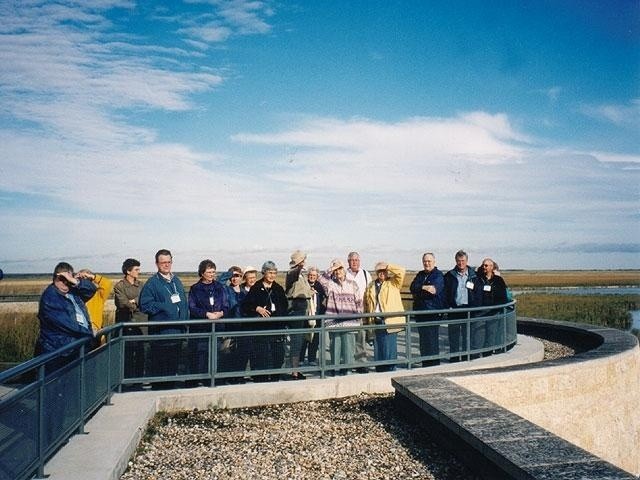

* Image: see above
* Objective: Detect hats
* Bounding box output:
[243,267,257,279]
[288,250,305,268]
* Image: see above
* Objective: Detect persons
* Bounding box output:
[189,259,230,386]
[410,253,445,366]
[316,258,363,377]
[138,249,188,389]
[73,269,113,348]
[299,267,326,366]
[346,252,377,373]
[445,249,513,363]
[114,259,145,387]
[242,261,288,381]
[285,249,316,380]
[365,262,407,371]
[217,266,249,384]
[33,262,97,374]
[240,270,258,295]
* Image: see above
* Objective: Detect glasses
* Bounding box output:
[232,274,242,278]
[481,264,491,268]
